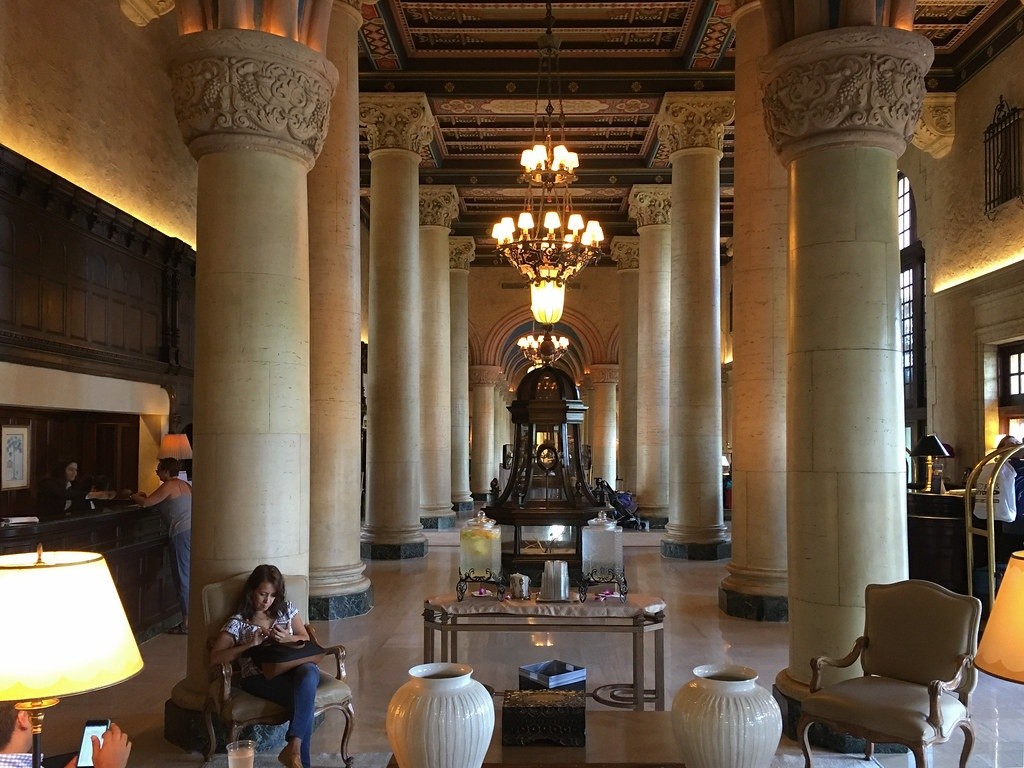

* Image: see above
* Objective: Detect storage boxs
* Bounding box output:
[500,689,587,748]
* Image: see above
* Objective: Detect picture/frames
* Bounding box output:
[2,424,32,490]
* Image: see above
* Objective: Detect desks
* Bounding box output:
[422,593,666,712]
[907,488,981,594]
[386,707,686,768]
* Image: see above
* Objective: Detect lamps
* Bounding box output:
[0,542,144,768]
[972,550,1023,685]
[157,434,193,461]
[490,2,604,365]
[910,434,950,493]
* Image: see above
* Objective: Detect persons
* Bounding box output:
[38,457,87,519]
[0,701,132,768]
[209,565,321,768]
[129,457,192,635]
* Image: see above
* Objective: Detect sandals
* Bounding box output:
[167,622,187,634]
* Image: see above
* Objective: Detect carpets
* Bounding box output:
[200,750,883,768]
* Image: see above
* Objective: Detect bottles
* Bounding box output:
[670,663,783,768]
[385,662,495,768]
[580,510,624,582]
[458,510,504,583]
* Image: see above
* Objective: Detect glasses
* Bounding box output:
[155,469,163,472]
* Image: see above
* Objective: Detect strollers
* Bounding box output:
[594,478,647,532]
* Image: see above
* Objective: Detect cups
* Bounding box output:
[226,740,256,768]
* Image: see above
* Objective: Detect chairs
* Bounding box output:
[199,571,354,768]
[796,578,983,768]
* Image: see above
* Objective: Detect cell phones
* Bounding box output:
[276,621,288,630]
[75,718,111,768]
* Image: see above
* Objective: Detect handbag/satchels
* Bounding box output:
[973,461,1016,522]
[85,475,117,508]
[242,628,327,681]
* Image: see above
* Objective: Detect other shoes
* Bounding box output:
[278,747,302,768]
